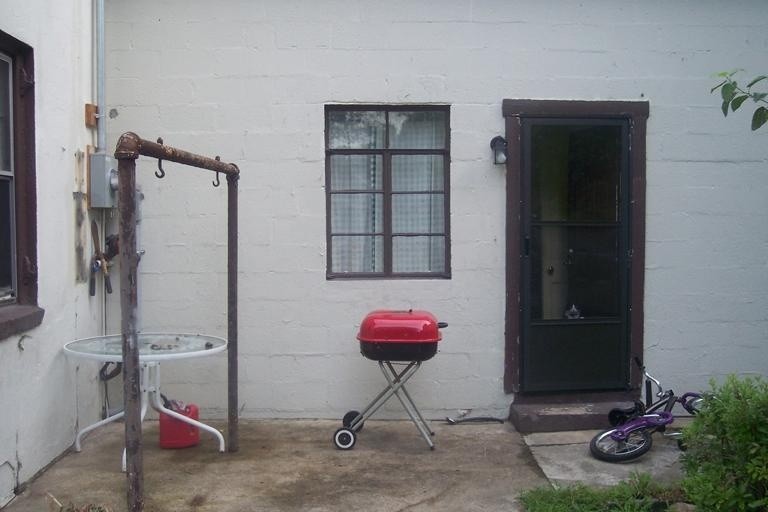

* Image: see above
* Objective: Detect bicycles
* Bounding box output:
[589,356,721,465]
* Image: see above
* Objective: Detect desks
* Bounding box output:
[64,330,228,472]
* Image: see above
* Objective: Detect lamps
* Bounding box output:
[491,135,511,165]
